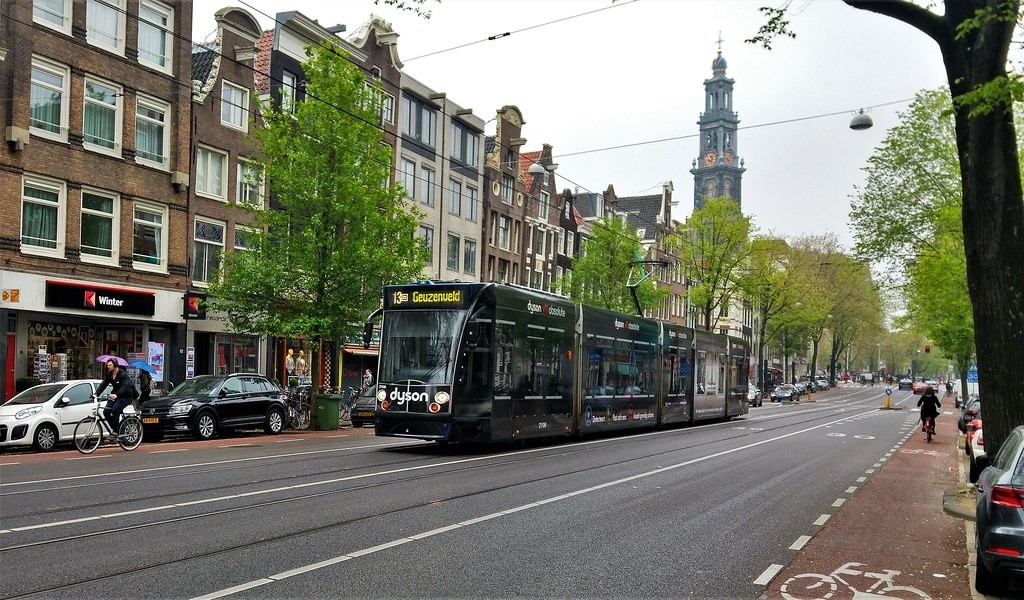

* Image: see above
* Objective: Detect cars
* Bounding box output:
[976,424,1023,596]
[350,384,376,428]
[770,375,831,403]
[957,394,987,484]
[0,379,137,454]
[747,385,763,407]
[898,377,938,394]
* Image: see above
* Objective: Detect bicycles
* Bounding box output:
[919,405,938,443]
[73,395,144,455]
[323,383,363,421]
[285,386,313,430]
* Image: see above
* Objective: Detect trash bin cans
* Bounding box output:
[314,393,343,429]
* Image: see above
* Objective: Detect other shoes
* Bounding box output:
[105,434,118,440]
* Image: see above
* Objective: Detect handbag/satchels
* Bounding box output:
[129,380,139,399]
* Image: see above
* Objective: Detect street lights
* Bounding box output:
[876,343,880,383]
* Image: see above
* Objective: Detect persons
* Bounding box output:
[946,381,952,397]
[773,375,781,387]
[138,368,152,404]
[286,349,295,377]
[921,376,925,382]
[697,382,704,394]
[363,369,374,391]
[917,386,940,435]
[90,358,135,439]
[295,350,309,377]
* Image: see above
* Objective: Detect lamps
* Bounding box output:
[528,163,544,175]
[547,163,559,173]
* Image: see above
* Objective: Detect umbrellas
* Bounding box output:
[96,355,130,366]
[128,360,157,374]
[763,367,783,382]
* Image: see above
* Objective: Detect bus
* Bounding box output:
[363,256,751,451]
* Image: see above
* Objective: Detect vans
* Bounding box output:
[950,380,979,409]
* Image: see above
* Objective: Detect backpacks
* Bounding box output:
[148,376,155,391]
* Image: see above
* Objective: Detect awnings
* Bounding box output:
[341,342,379,356]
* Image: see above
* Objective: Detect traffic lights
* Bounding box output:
[924,345,930,353]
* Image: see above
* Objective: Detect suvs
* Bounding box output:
[135,372,290,442]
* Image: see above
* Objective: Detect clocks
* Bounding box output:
[704,151,717,167]
[724,153,733,164]
